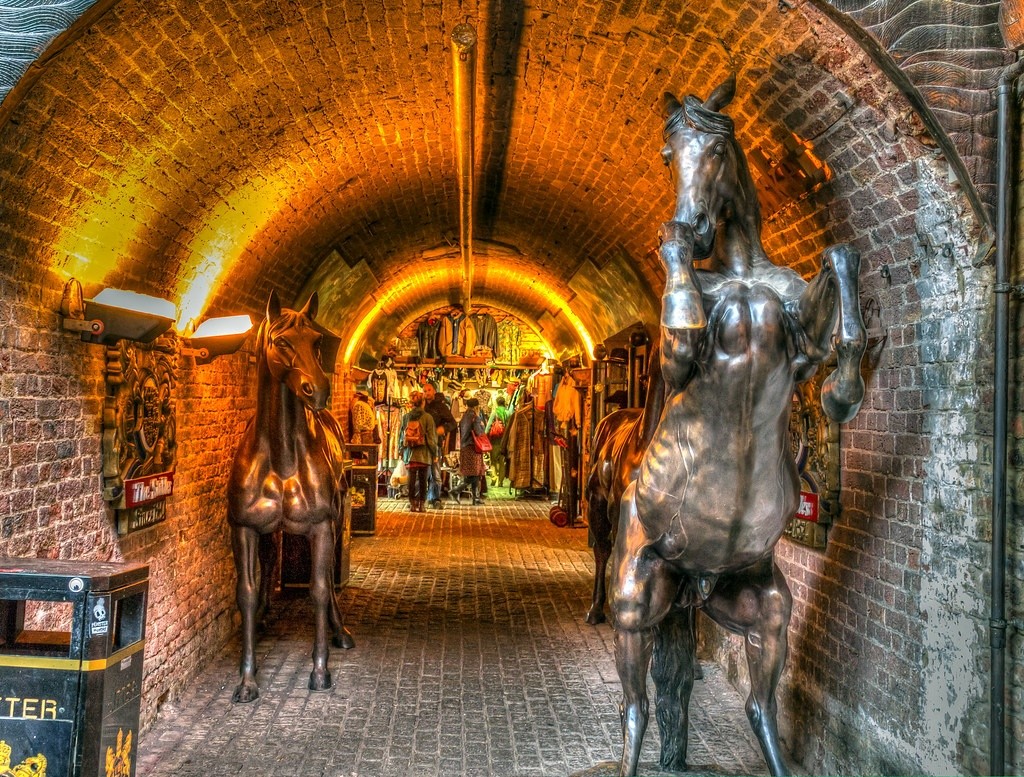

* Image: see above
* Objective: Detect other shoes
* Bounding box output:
[491,478,496,486]
[409,500,416,512]
[419,500,425,512]
[432,501,441,510]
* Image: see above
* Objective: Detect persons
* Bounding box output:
[423,383,456,509]
[475,388,491,414]
[399,391,439,512]
[349,391,382,445]
[448,398,486,505]
[486,396,509,487]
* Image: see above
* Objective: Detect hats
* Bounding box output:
[467,398,479,407]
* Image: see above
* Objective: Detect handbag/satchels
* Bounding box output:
[489,411,506,438]
[470,430,493,454]
[391,459,409,487]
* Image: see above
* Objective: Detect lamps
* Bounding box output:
[179,314,255,366]
[65,285,177,349]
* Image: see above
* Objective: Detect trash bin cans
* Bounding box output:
[281,460,352,589]
[345,443,379,536]
[0,555,150,777]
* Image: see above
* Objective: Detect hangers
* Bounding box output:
[429,313,438,320]
[452,309,461,314]
[564,367,569,377]
[478,307,485,314]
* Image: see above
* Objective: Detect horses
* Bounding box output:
[225,287,358,702]
[586,68,867,777]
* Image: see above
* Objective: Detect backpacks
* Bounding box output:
[403,414,426,448]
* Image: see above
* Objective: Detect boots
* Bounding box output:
[473,486,485,505]
[448,481,468,504]
